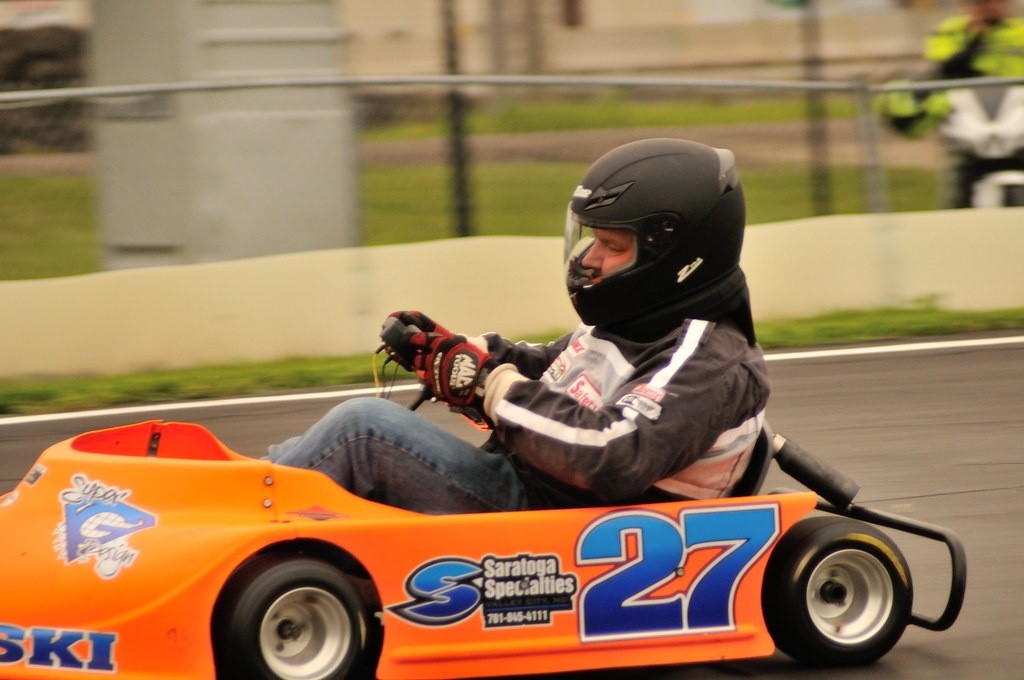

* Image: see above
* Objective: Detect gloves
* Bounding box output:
[379,310,488,393]
[408,331,502,431]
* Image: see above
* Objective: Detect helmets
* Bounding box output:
[563,137,746,326]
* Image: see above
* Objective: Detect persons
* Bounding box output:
[255,137,773,518]
[877,0,1024,211]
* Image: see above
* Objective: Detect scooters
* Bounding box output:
[905,80,1024,211]
